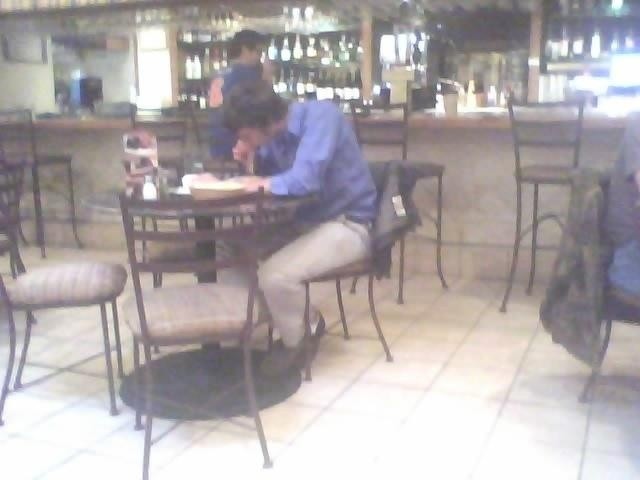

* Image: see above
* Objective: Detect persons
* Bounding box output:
[223,78,380,373]
[599,110,640,294]
[207,28,282,169]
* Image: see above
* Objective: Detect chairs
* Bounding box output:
[260,159,394,382]
[119,186,273,479]
[0,160,126,416]
[577,168,638,402]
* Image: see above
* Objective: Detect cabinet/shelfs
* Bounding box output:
[175,2,360,108]
[434,16,640,119]
[168,0,640,107]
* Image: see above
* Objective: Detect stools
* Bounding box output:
[499,102,585,313]
[129,106,259,263]
[3,109,83,258]
[350,105,449,305]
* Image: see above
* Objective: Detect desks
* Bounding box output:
[82,178,322,421]
